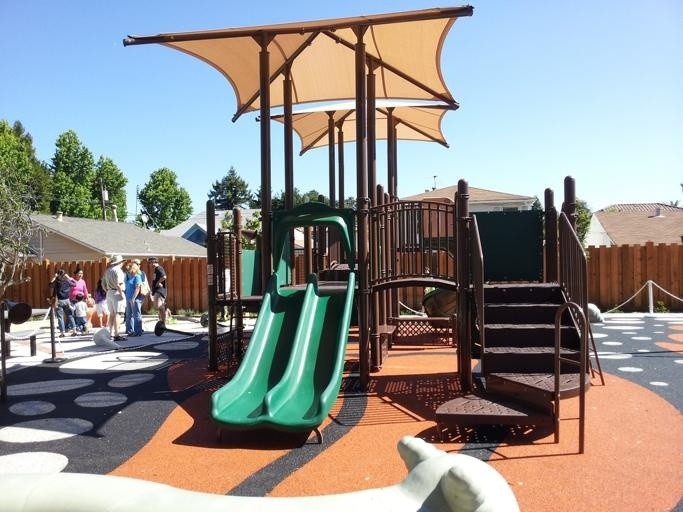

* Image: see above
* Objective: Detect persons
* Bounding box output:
[48,269,77,338]
[64,269,88,332]
[66,292,88,335]
[148,257,166,325]
[92,254,151,342]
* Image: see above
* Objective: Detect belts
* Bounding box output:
[107,288,121,291]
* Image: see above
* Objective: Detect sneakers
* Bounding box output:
[58,327,90,339]
[109,329,142,342]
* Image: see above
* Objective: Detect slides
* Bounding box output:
[210,271,356,429]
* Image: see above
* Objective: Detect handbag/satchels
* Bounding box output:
[51,295,61,309]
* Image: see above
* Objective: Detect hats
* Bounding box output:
[147,257,160,263]
[109,252,125,267]
